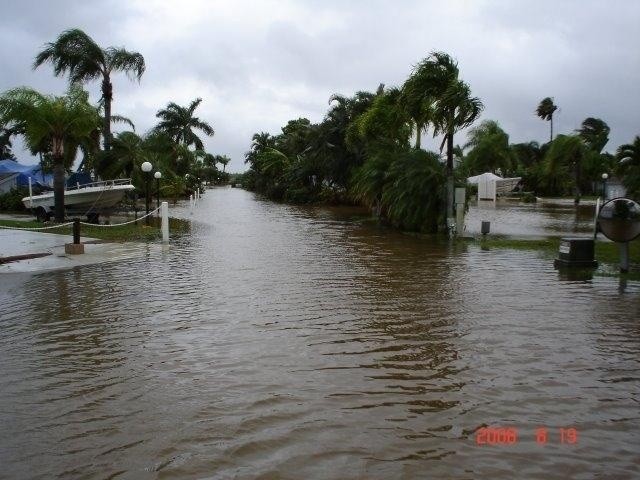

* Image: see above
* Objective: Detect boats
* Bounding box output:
[21,177,135,224]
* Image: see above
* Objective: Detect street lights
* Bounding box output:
[154,172,162,220]
[601,173,609,204]
[185,174,190,199]
[140,162,154,226]
